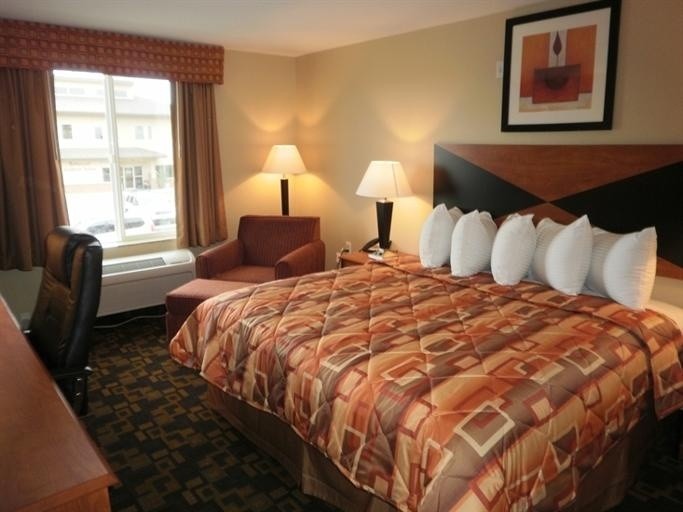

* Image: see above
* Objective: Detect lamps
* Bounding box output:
[262,145,308,217]
[355,160,413,253]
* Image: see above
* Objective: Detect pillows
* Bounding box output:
[419,203,659,314]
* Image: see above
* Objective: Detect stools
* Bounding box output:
[166,278,244,348]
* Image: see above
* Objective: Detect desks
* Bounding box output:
[1,293,121,510]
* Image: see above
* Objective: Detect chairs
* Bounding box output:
[195,215,325,283]
[23,225,103,416]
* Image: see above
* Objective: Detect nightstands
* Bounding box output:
[340,250,418,268]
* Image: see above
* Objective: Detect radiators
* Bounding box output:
[95,248,196,318]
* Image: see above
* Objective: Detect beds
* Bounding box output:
[208,143,682,512]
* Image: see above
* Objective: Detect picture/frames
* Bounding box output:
[500,1,622,133]
